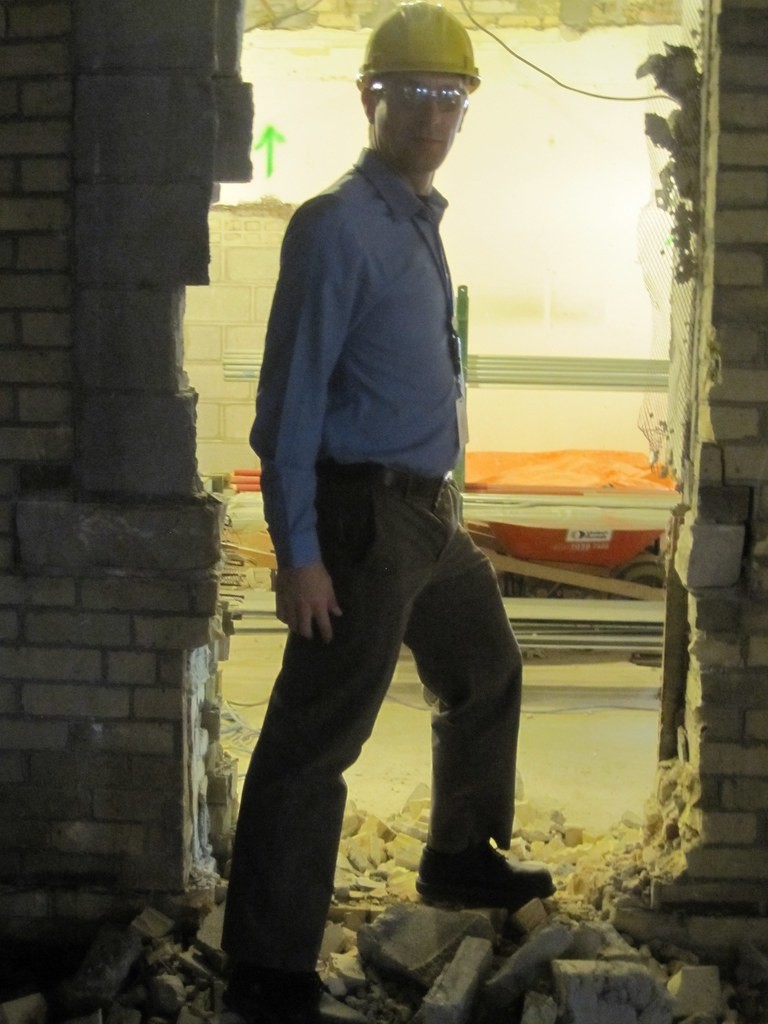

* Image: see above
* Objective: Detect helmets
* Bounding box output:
[363,4,480,93]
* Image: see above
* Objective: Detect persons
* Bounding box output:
[222,2,556,1024]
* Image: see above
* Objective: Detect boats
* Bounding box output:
[227,471,681,580]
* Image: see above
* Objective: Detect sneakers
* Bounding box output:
[415,841,556,905]
[221,963,322,1024]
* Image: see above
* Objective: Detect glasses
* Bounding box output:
[369,81,470,112]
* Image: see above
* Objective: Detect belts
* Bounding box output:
[317,460,442,498]
